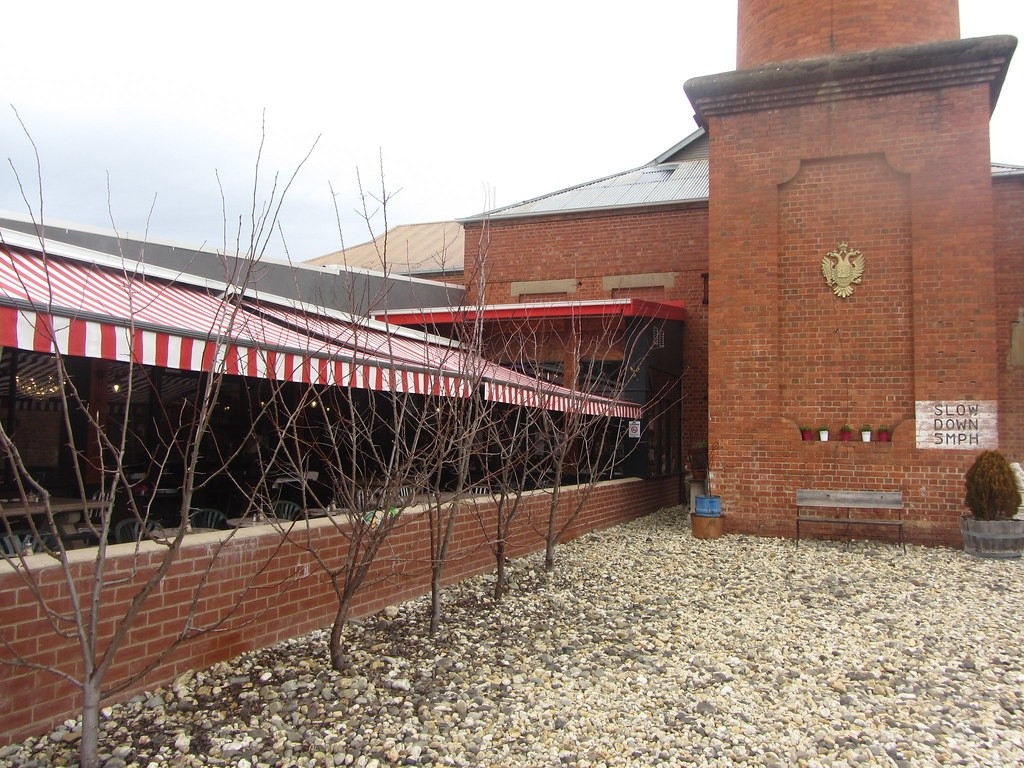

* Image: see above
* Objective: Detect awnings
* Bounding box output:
[0,243,471,397]
[233,299,643,418]
[368,297,684,326]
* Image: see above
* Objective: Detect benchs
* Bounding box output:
[795,490,907,555]
[54,531,112,545]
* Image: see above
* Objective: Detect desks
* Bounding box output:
[390,492,479,506]
[0,498,115,552]
[226,517,292,528]
[306,508,351,516]
[149,527,220,541]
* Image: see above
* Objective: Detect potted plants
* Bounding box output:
[689,439,708,479]
[801,426,813,441]
[840,425,854,441]
[876,424,891,442]
[815,424,832,441]
[695,442,721,517]
[860,425,874,442]
[958,449,1024,558]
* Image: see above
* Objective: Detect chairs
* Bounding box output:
[190,509,233,530]
[333,485,412,508]
[472,485,491,494]
[270,500,306,521]
[113,518,163,545]
